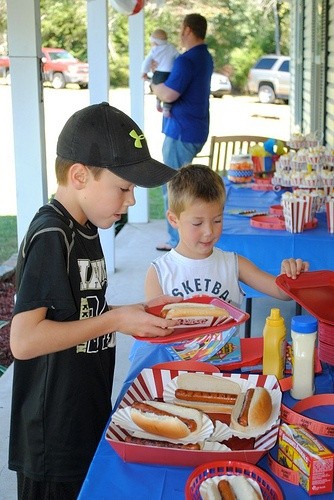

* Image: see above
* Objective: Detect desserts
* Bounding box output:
[271,132,334,187]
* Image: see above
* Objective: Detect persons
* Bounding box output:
[145,164,309,338]
[7,102,184,500]
[150,14,214,251]
[140,30,182,117]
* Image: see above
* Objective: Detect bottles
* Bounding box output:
[227,155,254,183]
[290,316,317,400]
[263,307,287,380]
[250,139,285,173]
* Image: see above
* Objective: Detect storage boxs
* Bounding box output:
[104,368,282,467]
[275,424,334,495]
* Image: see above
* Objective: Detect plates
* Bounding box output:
[129,296,250,344]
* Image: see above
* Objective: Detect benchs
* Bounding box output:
[207,135,289,177]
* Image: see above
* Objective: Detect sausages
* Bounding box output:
[124,435,200,451]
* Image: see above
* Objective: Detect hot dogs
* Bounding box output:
[231,387,272,431]
[174,372,242,414]
[205,474,261,500]
[160,303,229,319]
[131,401,203,437]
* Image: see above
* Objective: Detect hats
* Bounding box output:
[56,102,179,189]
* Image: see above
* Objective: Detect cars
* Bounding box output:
[210,71,232,99]
[247,53,292,104]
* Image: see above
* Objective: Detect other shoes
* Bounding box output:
[155,244,171,251]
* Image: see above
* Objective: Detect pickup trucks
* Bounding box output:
[0,47,88,90]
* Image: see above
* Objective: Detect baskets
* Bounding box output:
[184,460,284,500]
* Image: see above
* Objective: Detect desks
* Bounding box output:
[75,336,334,500]
[218,175,334,339]
